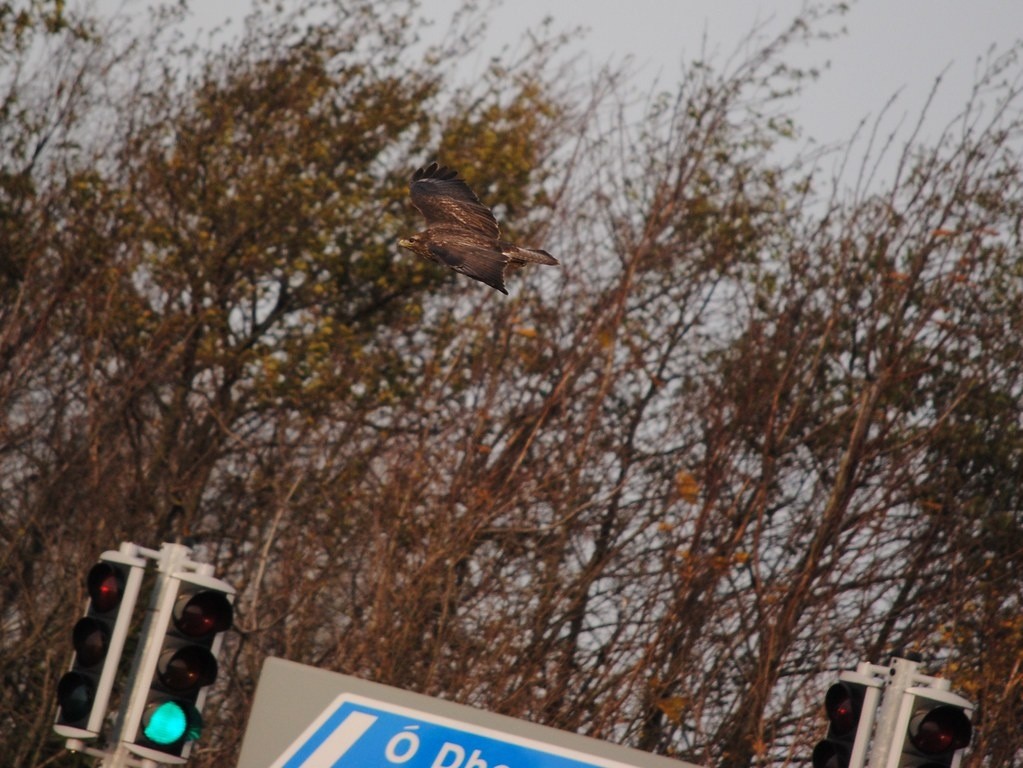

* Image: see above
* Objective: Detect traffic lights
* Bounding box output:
[122,570,239,764]
[51,549,148,739]
[809,671,884,768]
[884,688,977,768]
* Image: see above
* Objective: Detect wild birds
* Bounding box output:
[397,162,561,298]
